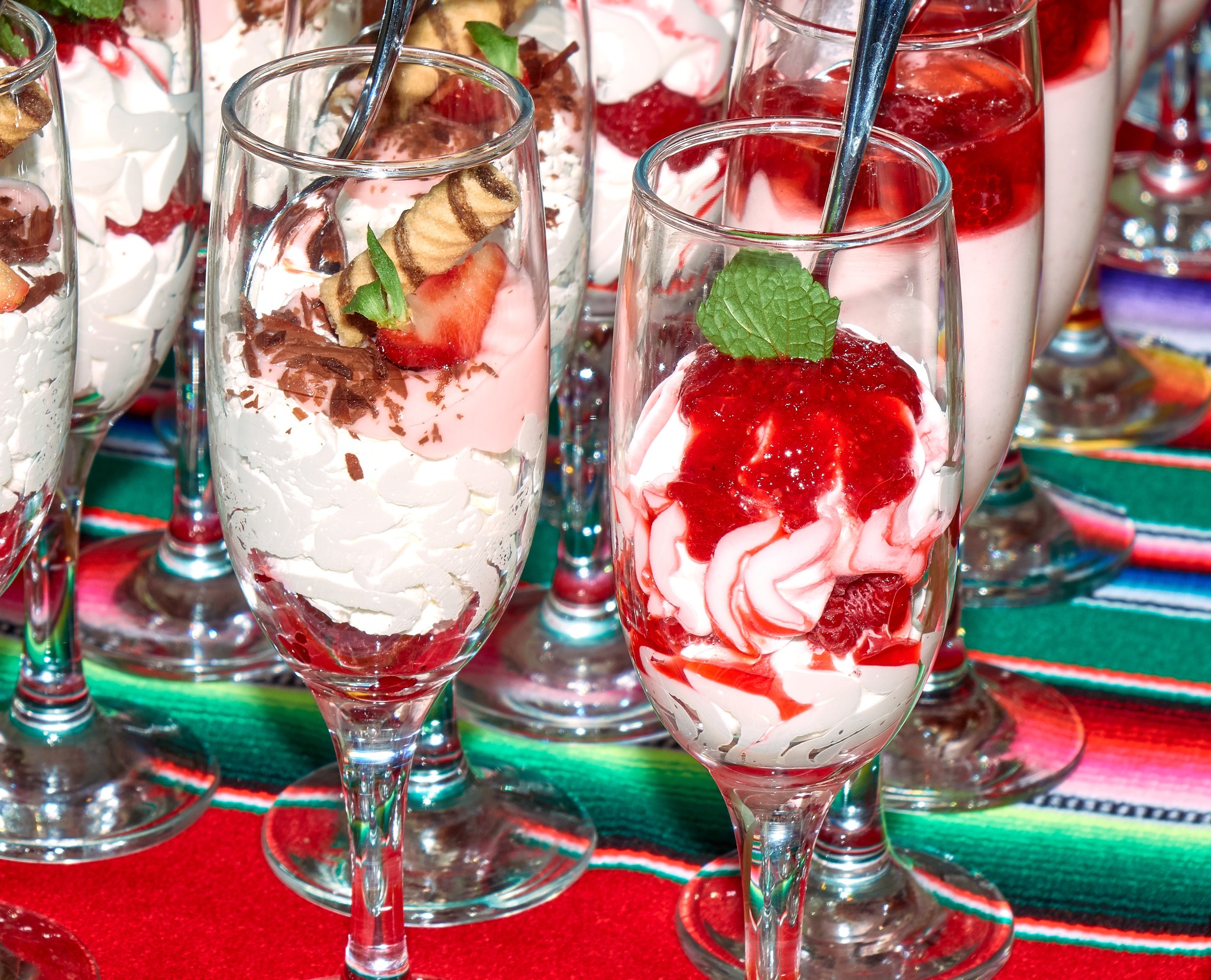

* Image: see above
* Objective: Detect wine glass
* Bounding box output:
[0,0,1211,980]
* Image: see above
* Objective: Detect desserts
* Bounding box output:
[0,0,1191,788]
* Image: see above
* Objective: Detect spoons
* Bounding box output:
[243,0,414,329]
